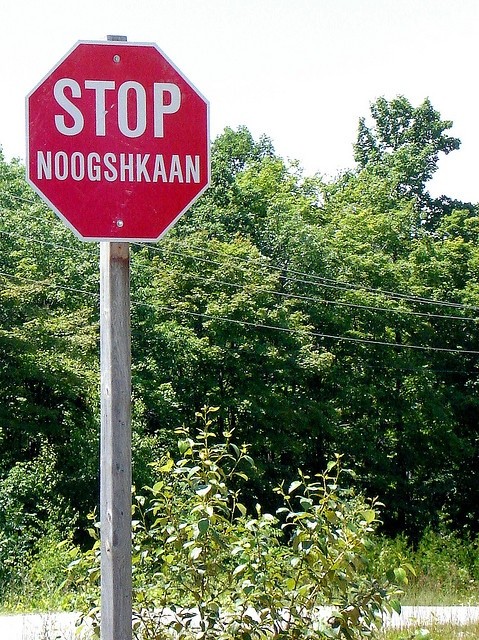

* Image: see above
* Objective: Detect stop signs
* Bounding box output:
[25,39,211,244]
